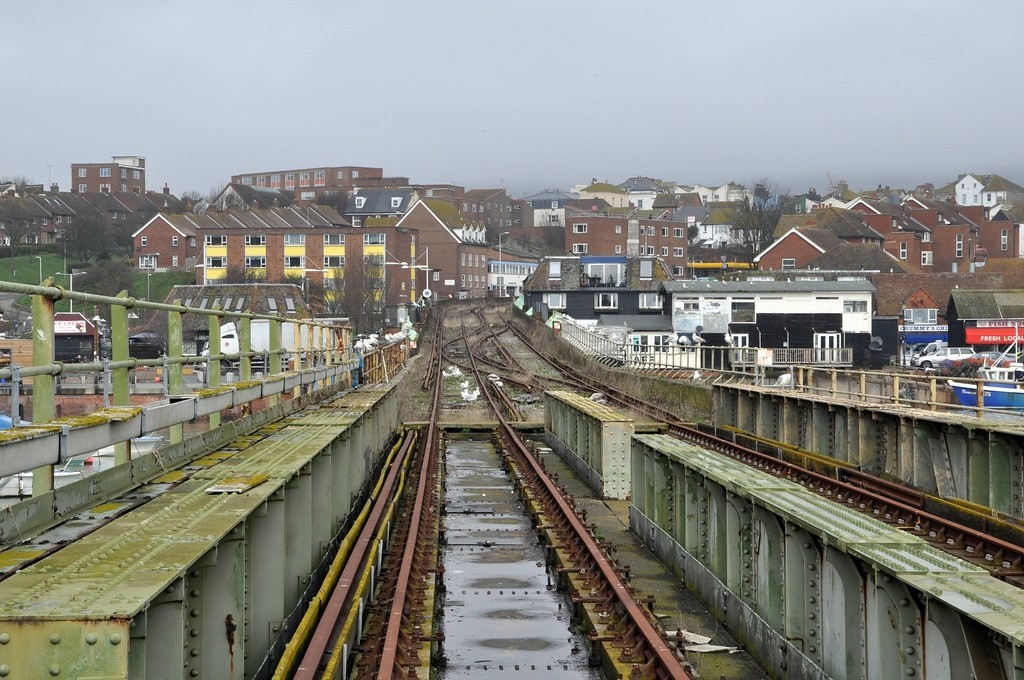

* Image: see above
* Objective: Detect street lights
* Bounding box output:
[380,262,408,266]
[148,253,160,301]
[56,272,86,312]
[401,247,428,289]
[899,301,907,367]
[36,256,42,283]
[302,252,329,291]
[499,232,509,297]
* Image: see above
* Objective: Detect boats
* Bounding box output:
[947,321,1024,409]
[92,436,163,457]
[0,472,81,496]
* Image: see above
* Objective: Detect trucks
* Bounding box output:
[910,343,940,366]
[202,318,350,374]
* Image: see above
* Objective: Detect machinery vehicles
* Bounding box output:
[379,302,420,338]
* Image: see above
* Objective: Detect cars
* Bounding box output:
[914,348,977,368]
[968,351,1014,368]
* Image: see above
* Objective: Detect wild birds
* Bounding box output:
[680,263,820,289]
[561,312,621,344]
[353,315,413,354]
[589,391,607,405]
[725,332,737,347]
[693,370,702,382]
[774,373,791,388]
[663,330,706,347]
[442,367,480,403]
